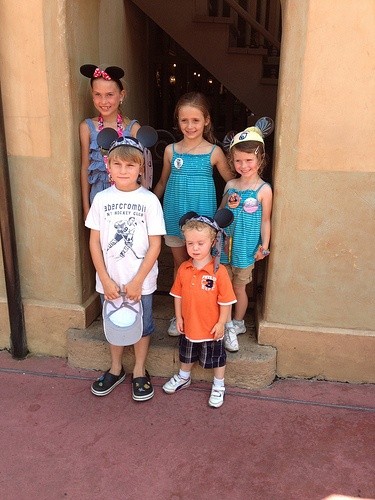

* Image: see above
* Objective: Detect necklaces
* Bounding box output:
[174,139,204,169]
[98,110,123,187]
[227,177,258,208]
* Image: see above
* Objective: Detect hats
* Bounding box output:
[222,116,274,150]
[97,124,158,154]
[101,285,144,346]
[177,208,233,238]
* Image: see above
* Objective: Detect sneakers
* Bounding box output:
[162,373,192,395]
[208,382,226,407]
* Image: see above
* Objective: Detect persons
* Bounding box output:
[162,207,237,408]
[216,117,274,335]
[85,126,167,401]
[79,64,141,221]
[154,91,239,352]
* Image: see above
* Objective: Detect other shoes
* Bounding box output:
[233,320,247,336]
[167,316,182,337]
[223,326,240,353]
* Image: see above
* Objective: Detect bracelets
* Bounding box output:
[257,244,270,256]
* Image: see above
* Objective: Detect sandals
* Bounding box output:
[131,369,155,402]
[90,363,126,397]
